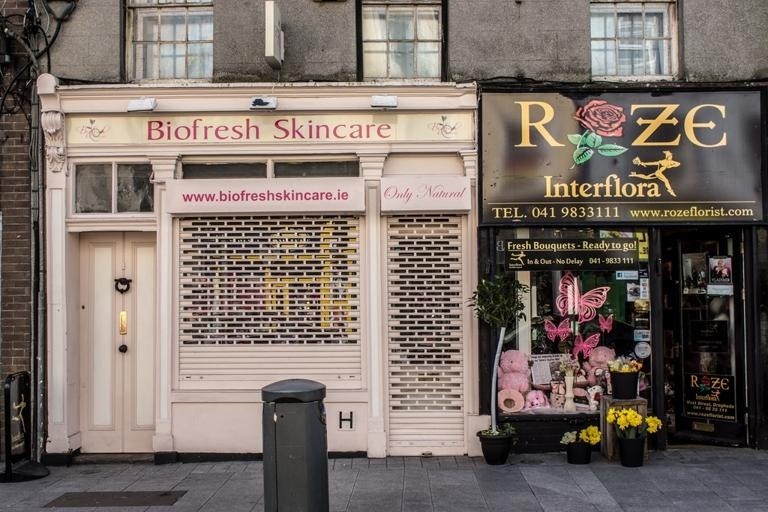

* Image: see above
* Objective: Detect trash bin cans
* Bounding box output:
[261,379,329,512]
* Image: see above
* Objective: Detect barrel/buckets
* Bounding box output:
[618,438,646,468]
[610,370,639,399]
[567,441,591,464]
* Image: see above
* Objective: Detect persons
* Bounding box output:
[712,258,731,282]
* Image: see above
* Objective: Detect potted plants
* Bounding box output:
[464,272,530,466]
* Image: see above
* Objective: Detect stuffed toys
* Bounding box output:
[498,346,615,412]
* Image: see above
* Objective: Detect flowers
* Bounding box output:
[560,425,602,445]
[606,407,663,438]
[565,100,630,169]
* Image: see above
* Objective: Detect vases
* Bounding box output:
[567,442,592,463]
[616,436,645,467]
